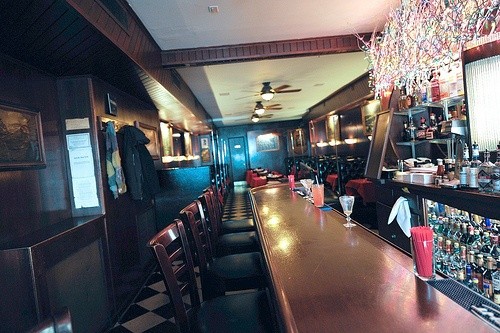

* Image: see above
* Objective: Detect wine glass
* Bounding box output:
[300,179,315,199]
[339,196,355,228]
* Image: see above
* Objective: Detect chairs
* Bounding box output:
[146,167,284,333]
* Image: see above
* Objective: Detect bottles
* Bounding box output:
[436,143,500,198]
[399,67,467,141]
[427,200,500,306]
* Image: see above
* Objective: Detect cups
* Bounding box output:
[311,184,324,207]
[289,175,295,191]
[410,237,436,282]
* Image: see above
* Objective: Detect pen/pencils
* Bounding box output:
[400,160,403,172]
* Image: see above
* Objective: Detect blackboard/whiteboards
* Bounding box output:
[364,110,393,179]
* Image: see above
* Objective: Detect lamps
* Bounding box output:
[251,114,259,123]
[260,82,276,101]
[255,101,265,114]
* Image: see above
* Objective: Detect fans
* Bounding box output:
[235,114,273,122]
[226,101,283,115]
[234,82,302,100]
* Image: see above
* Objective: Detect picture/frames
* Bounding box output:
[0,102,47,172]
[135,121,160,160]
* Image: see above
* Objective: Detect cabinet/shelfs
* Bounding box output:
[376,97,500,308]
